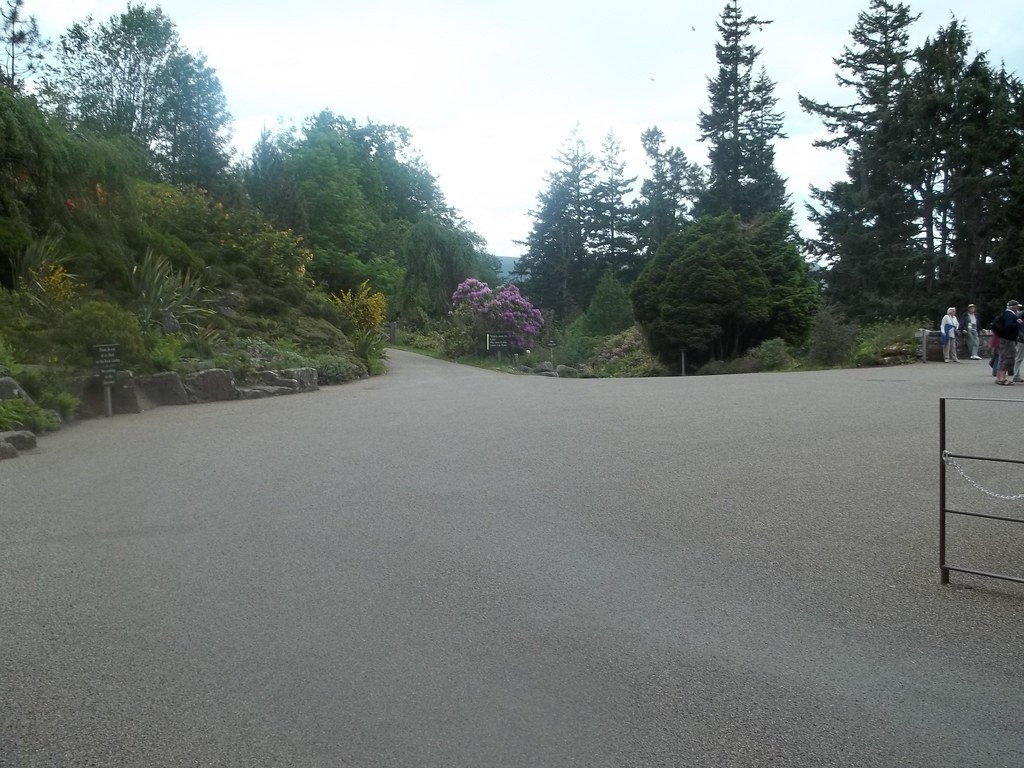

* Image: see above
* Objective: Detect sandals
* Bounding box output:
[998,379,1015,385]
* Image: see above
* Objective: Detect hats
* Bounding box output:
[1007,300,1022,307]
[968,304,976,308]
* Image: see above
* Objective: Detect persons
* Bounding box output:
[989,300,1024,385]
[960,304,985,360]
[940,307,961,363]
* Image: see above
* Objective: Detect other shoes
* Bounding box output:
[953,360,960,362]
[970,355,982,359]
[944,359,949,362]
